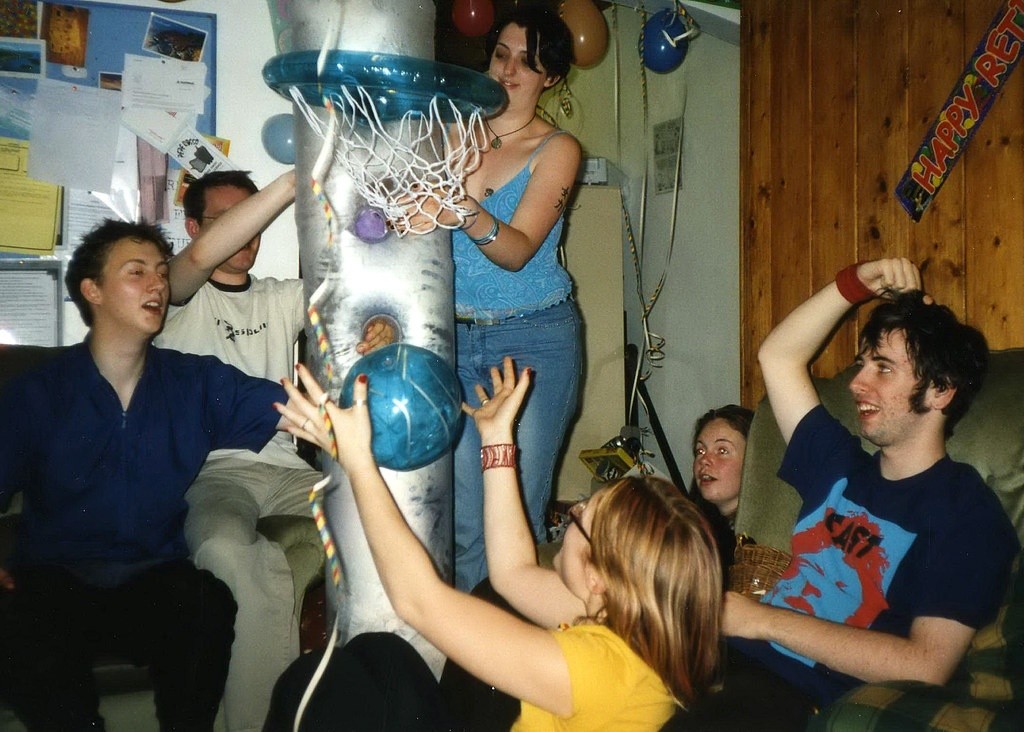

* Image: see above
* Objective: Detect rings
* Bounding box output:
[352,399,369,405]
[302,418,311,430]
[481,399,490,407]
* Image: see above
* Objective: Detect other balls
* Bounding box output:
[340,342,464,471]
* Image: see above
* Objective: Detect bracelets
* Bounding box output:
[459,201,478,230]
[470,215,499,245]
[480,444,517,473]
[835,260,876,306]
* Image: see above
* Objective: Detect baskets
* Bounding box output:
[730,544,792,603]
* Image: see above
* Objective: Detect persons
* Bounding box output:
[395,13,583,594]
[0,171,324,732]
[271,357,722,732]
[659,257,1018,732]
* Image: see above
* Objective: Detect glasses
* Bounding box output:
[568,499,591,544]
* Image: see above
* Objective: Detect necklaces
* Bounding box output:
[484,110,537,149]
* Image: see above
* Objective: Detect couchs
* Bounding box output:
[0,516,326,732]
[707,347,1024,732]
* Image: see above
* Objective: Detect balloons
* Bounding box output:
[261,113,296,164]
[452,0,494,38]
[339,343,463,474]
[558,0,608,67]
[638,10,689,75]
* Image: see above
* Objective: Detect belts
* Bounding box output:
[455,318,498,325]
